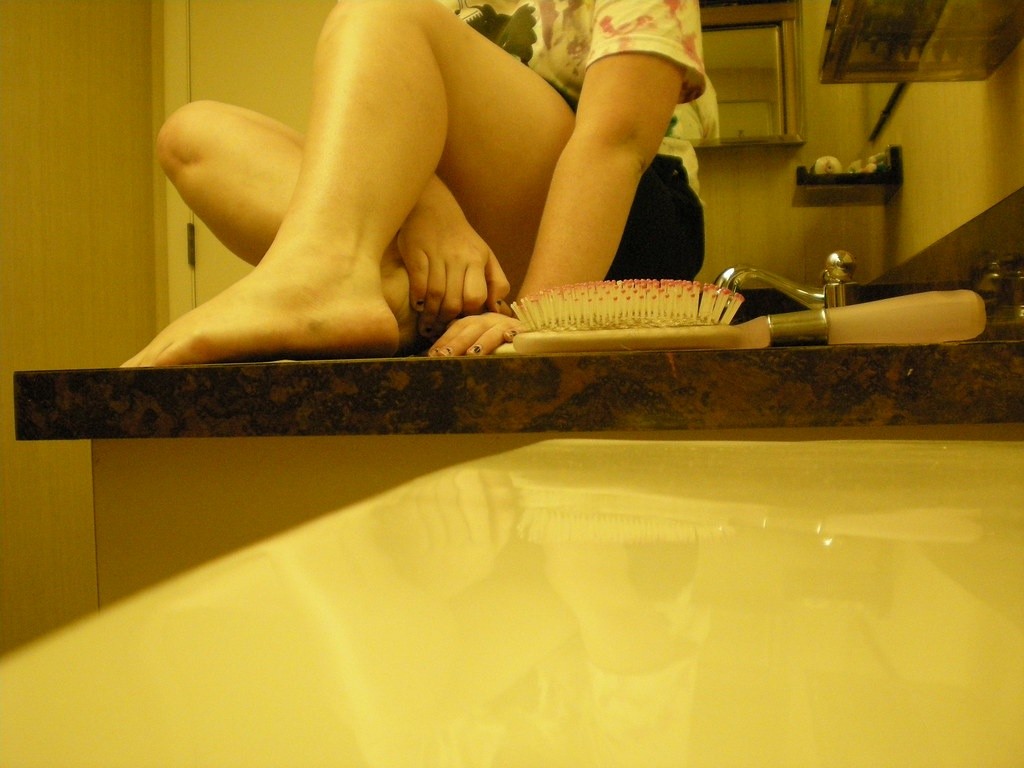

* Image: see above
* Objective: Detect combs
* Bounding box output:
[510,278,987,355]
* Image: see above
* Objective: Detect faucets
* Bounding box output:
[714,249,855,310]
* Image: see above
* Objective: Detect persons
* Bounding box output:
[119,0,705,369]
